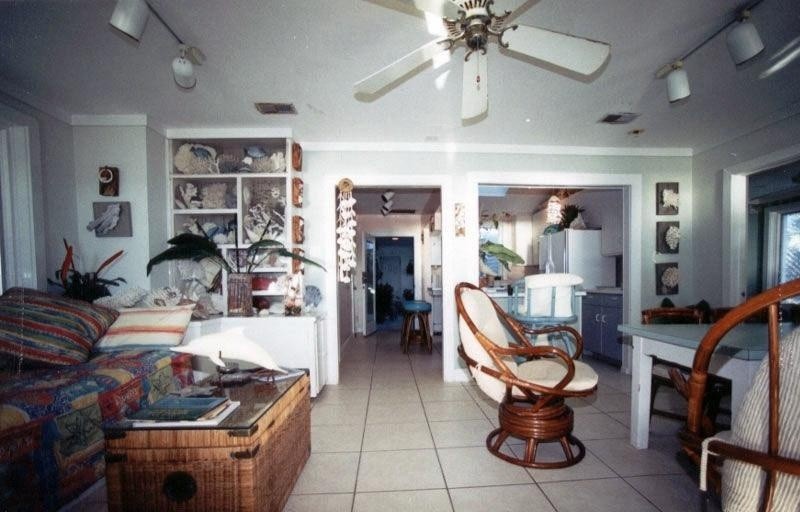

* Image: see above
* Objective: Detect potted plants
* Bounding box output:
[146,217,328,316]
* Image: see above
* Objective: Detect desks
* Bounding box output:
[106,367,313,512]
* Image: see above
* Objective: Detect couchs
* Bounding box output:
[0,346,195,511]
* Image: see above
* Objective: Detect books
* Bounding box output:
[120,392,242,427]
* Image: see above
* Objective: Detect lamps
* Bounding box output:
[547,187,563,225]
[111,1,206,89]
[381,189,395,216]
[657,0,766,103]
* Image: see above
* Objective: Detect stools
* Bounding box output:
[401,300,432,354]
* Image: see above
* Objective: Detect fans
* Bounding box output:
[352,1,612,123]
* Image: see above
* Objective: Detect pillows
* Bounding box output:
[459,288,516,402]
[0,287,120,368]
[524,274,583,316]
[93,303,196,347]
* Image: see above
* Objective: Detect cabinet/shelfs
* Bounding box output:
[166,125,293,313]
[537,228,617,291]
[581,293,622,366]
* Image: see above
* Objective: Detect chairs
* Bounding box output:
[713,304,735,434]
[451,283,600,470]
[643,307,702,427]
[507,284,583,359]
[673,277,800,511]
